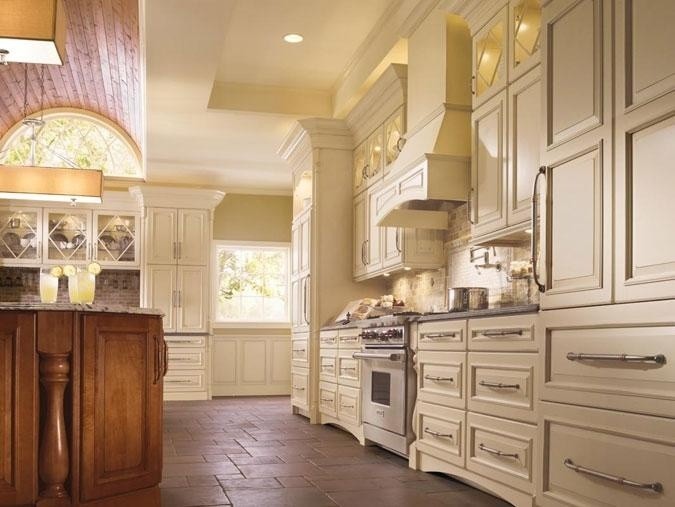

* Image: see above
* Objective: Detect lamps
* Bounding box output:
[0,0,68,66]
[0,118,104,204]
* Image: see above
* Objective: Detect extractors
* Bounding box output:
[368,110,470,230]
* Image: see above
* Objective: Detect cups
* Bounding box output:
[38,265,60,304]
[67,265,95,303]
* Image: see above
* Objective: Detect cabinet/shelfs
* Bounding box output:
[470,1,541,109]
[291,334,316,425]
[539,2,673,308]
[141,201,214,267]
[0,309,40,507]
[0,197,44,268]
[44,200,142,272]
[414,322,539,495]
[268,331,291,396]
[341,60,445,282]
[141,262,214,400]
[290,272,311,333]
[470,63,542,238]
[213,331,269,398]
[77,312,169,504]
[538,307,673,504]
[321,330,363,445]
[290,204,314,272]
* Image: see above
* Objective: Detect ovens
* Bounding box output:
[354,348,415,460]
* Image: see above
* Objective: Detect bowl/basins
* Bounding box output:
[446,287,489,311]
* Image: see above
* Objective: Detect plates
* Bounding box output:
[1,232,135,251]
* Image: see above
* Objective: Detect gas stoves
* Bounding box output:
[358,313,448,343]
